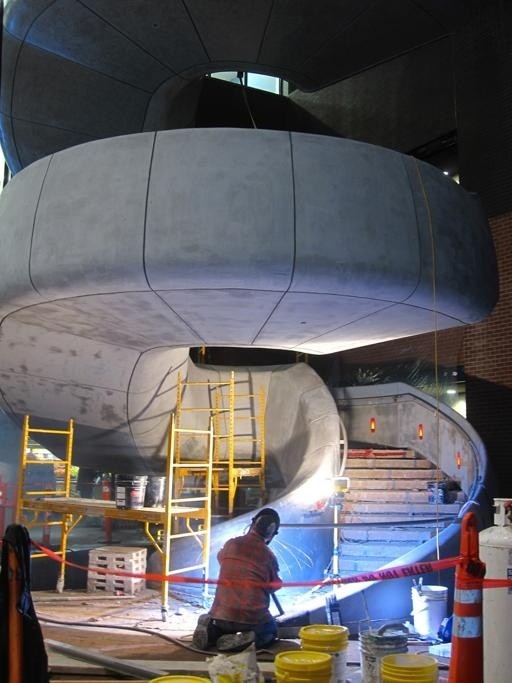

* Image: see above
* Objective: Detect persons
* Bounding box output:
[191,508,284,655]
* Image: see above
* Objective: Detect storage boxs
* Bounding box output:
[87,546,148,594]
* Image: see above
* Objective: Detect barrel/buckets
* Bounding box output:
[427,481,446,505]
[358,629,408,683]
[149,675,212,683]
[410,585,449,635]
[114,474,147,509]
[144,476,166,507]
[299,624,349,683]
[380,654,438,683]
[275,650,331,683]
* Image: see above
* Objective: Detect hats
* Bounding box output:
[252,508,280,545]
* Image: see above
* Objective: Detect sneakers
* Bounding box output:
[193,614,213,650]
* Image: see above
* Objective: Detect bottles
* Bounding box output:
[102,478,111,500]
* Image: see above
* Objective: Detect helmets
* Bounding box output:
[216,631,256,651]
